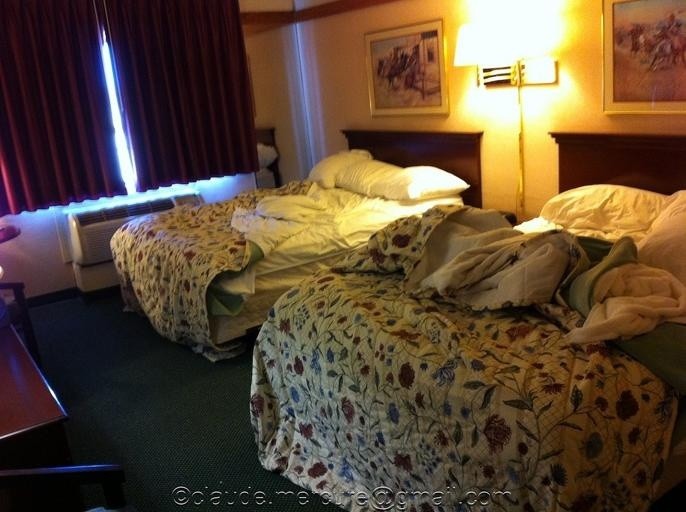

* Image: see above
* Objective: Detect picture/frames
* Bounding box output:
[597,0,686,116]
[361,16,449,119]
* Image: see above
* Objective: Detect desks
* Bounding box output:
[0,315,74,470]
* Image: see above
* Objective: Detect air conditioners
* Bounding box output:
[61,191,206,294]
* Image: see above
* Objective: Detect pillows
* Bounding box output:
[307,146,370,190]
[371,164,469,204]
[542,177,666,236]
[336,156,400,197]
[633,184,685,284]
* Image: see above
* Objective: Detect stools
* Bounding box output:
[0,280,47,367]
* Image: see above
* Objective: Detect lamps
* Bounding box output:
[452,18,536,225]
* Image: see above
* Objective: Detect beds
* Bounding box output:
[247,130,685,511]
[108,129,485,365]
